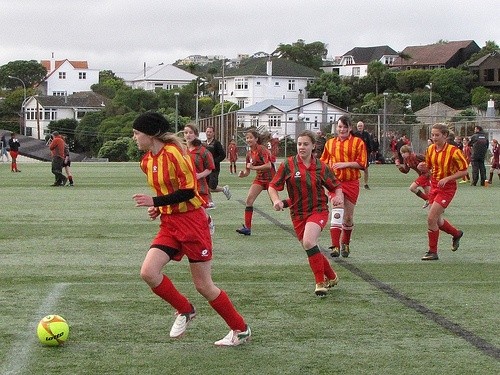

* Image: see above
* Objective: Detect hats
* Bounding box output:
[133,113,170,137]
[53,131,59,136]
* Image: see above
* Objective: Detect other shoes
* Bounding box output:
[422,200,429,208]
[364,185,370,190]
[63,178,68,186]
[459,180,466,184]
[208,216,215,235]
[11,170,14,172]
[15,170,21,172]
[485,180,492,184]
[469,183,476,186]
[51,184,59,186]
[68,182,73,186]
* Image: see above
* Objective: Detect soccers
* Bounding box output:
[36,314,69,346]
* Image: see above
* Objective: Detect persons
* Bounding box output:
[267,130,343,296]
[49,131,73,186]
[353,121,373,189]
[446,126,489,186]
[132,112,251,346]
[321,116,367,257]
[395,145,431,208]
[369,133,385,163]
[265,134,276,155]
[486,139,500,183]
[203,127,231,200]
[0,136,10,161]
[314,131,326,158]
[390,135,411,166]
[184,125,215,235]
[229,139,239,173]
[428,139,434,145]
[236,130,276,235]
[416,123,469,259]
[9,133,21,172]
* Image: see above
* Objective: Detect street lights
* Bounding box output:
[8,76,28,136]
[426,83,432,123]
[195,79,204,131]
[383,92,389,158]
[174,92,180,134]
[220,57,233,148]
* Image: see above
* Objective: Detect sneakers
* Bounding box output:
[208,202,215,209]
[224,185,231,200]
[214,326,251,346]
[328,246,340,257]
[341,243,350,258]
[315,282,328,296]
[169,304,197,338]
[324,273,339,287]
[421,251,438,260]
[236,223,251,235]
[452,230,463,251]
